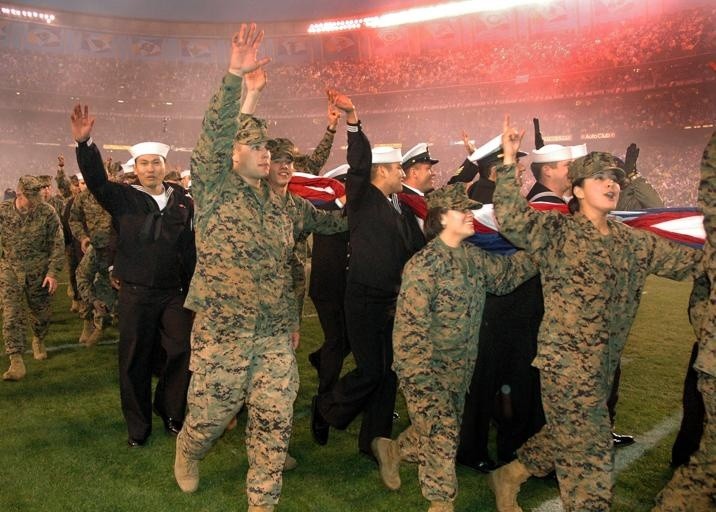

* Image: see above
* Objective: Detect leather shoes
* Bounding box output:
[393,412,400,422]
[152,402,183,437]
[310,395,330,445]
[358,434,378,464]
[309,341,325,379]
[128,433,148,447]
[544,470,559,488]
[612,433,636,448]
[671,459,687,470]
[456,456,503,474]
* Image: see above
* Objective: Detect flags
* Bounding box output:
[287,172,706,250]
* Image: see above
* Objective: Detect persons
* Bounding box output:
[0,175,66,381]
[250,48,309,156]
[308,164,351,399]
[607,143,667,447]
[239,66,349,473]
[35,150,120,347]
[311,87,413,471]
[651,133,716,512]
[392,142,439,418]
[0,50,228,191]
[291,101,343,325]
[174,23,309,512]
[310,48,457,189]
[370,182,539,512]
[71,104,196,447]
[457,8,715,207]
[496,142,587,487]
[456,134,528,472]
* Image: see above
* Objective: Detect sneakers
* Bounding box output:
[67,284,75,298]
[3,353,26,381]
[427,500,455,512]
[70,299,79,313]
[32,335,48,361]
[370,437,401,491]
[86,329,104,347]
[248,505,274,512]
[283,453,297,471]
[487,459,532,512]
[228,415,237,430]
[78,319,94,343]
[174,431,200,494]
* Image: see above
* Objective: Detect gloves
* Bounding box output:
[533,118,544,151]
[624,143,639,180]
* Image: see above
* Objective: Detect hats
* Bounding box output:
[232,113,278,150]
[469,131,529,168]
[180,170,190,178]
[265,137,294,161]
[371,147,403,164]
[109,163,120,176]
[18,175,45,206]
[425,181,482,211]
[531,144,572,164]
[74,173,84,180]
[121,158,135,173]
[323,163,350,181]
[35,176,53,187]
[563,143,589,158]
[401,142,439,170]
[127,142,170,160]
[566,152,626,186]
[164,170,181,180]
[4,188,16,200]
